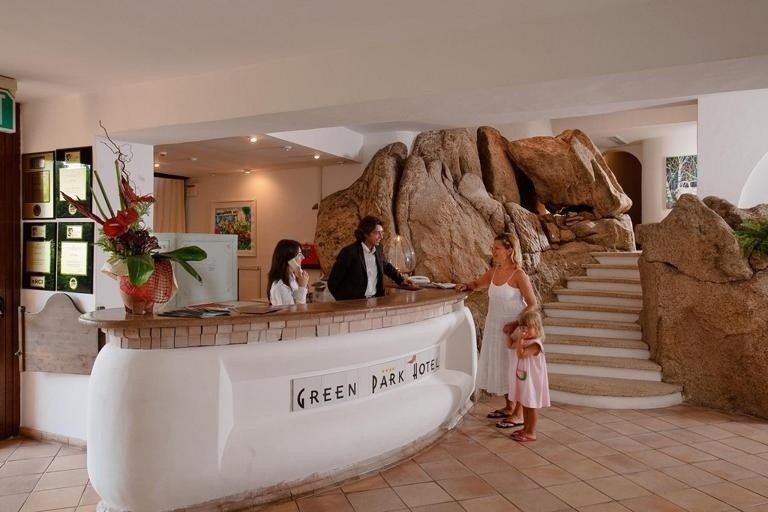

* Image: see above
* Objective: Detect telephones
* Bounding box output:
[288,258,304,278]
[408,276,430,284]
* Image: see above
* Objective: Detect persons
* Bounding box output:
[326,216,415,301]
[266,239,309,306]
[506,312,551,441]
[455,233,537,428]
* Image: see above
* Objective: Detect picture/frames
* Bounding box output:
[212,200,259,258]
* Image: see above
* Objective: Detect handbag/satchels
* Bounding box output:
[515,368,527,380]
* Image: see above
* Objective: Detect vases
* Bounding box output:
[120,275,155,314]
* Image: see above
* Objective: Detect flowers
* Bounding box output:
[58,116,210,302]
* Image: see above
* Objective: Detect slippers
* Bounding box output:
[496,418,524,428]
[487,409,512,418]
[511,429,536,441]
